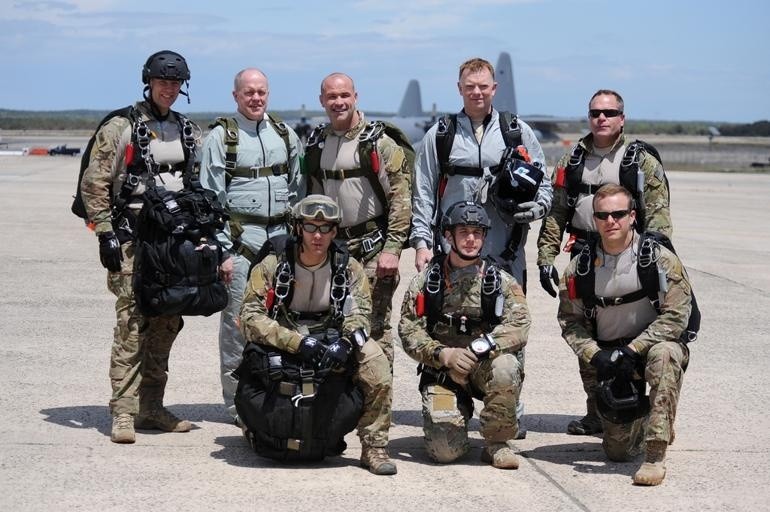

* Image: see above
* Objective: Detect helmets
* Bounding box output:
[592,371,651,424]
[441,199,493,239]
[491,159,545,217]
[141,50,191,83]
[291,194,344,237]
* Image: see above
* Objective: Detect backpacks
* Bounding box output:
[69,106,198,218]
[231,340,366,468]
[131,186,229,318]
[576,233,701,344]
[246,232,362,317]
[306,120,416,250]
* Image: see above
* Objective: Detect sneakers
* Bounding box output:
[568,413,604,436]
[111,412,137,443]
[516,419,527,439]
[480,441,520,469]
[134,404,193,432]
[633,445,668,486]
[360,444,398,475]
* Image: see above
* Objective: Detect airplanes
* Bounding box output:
[279,53,541,149]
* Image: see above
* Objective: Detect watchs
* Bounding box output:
[352,328,367,352]
[469,338,490,360]
[609,348,623,364]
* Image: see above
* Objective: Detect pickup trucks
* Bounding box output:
[48,145,81,156]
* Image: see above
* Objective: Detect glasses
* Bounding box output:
[593,209,632,221]
[588,108,624,119]
[300,219,337,234]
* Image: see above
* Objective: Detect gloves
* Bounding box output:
[318,338,354,372]
[607,345,641,380]
[446,368,470,385]
[299,335,327,365]
[98,233,125,273]
[590,350,613,381]
[539,263,560,299]
[438,347,478,375]
[512,200,547,225]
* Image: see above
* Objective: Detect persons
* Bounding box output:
[397,198,533,473]
[293,115,312,154]
[556,182,694,486]
[300,70,417,453]
[197,67,306,419]
[234,193,398,476]
[72,48,205,443]
[409,57,554,441]
[535,89,673,435]
